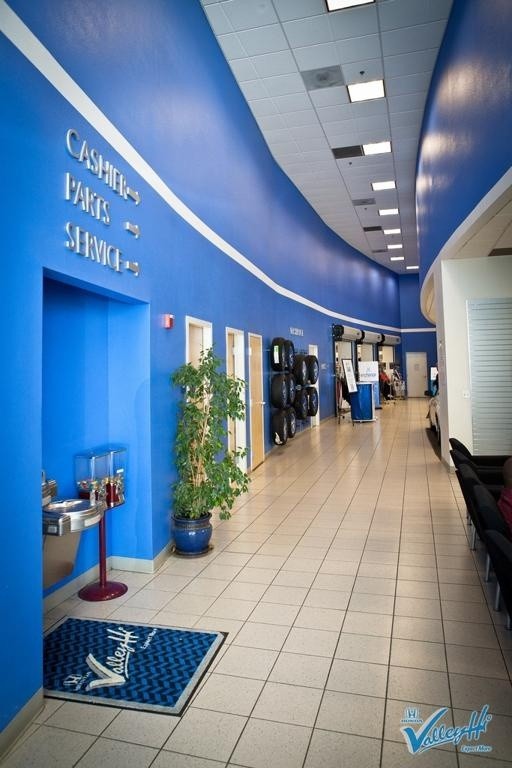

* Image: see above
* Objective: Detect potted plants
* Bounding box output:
[172,342,252,557]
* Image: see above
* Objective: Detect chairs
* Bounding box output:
[448,438,512,632]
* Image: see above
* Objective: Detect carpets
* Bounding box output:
[44,614,229,717]
